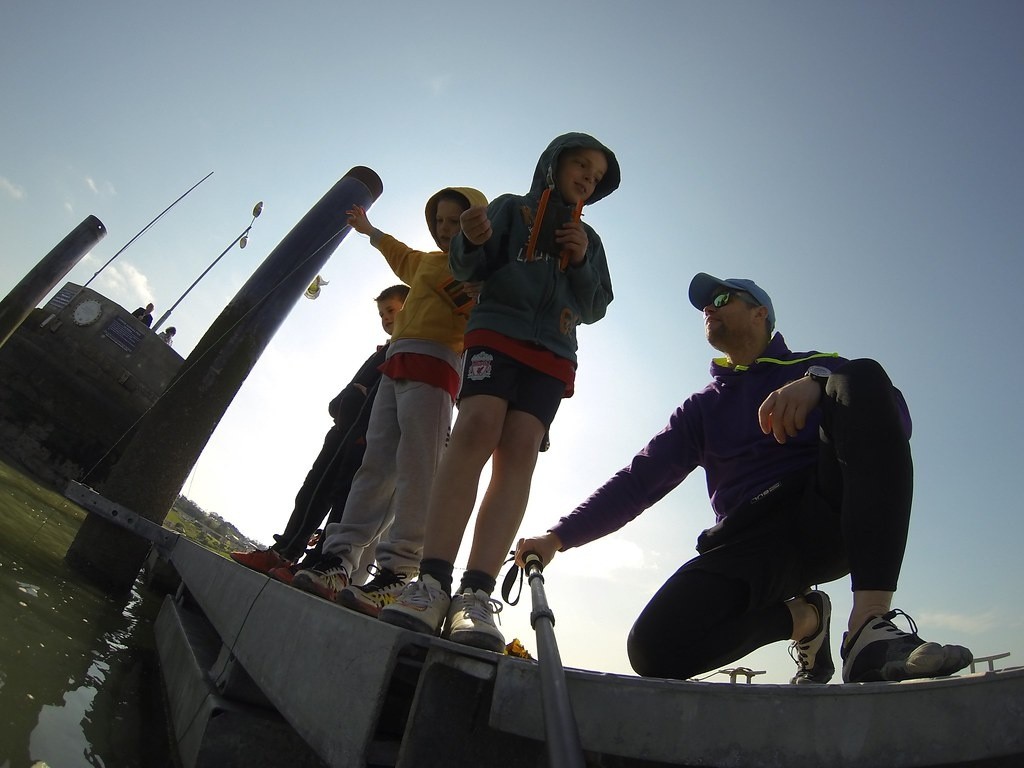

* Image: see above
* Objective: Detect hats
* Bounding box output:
[688,272,776,331]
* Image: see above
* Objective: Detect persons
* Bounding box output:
[514,273,975,684]
[293,186,489,618]
[374,132,622,651]
[229,285,410,586]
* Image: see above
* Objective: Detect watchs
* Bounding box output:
[803,364,831,383]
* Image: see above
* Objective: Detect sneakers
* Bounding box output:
[335,564,411,617]
[441,587,507,653]
[840,610,973,685]
[268,562,306,583]
[230,548,290,574]
[294,552,353,600]
[788,591,836,685]
[379,574,451,634]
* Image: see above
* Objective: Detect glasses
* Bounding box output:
[702,291,731,319]
